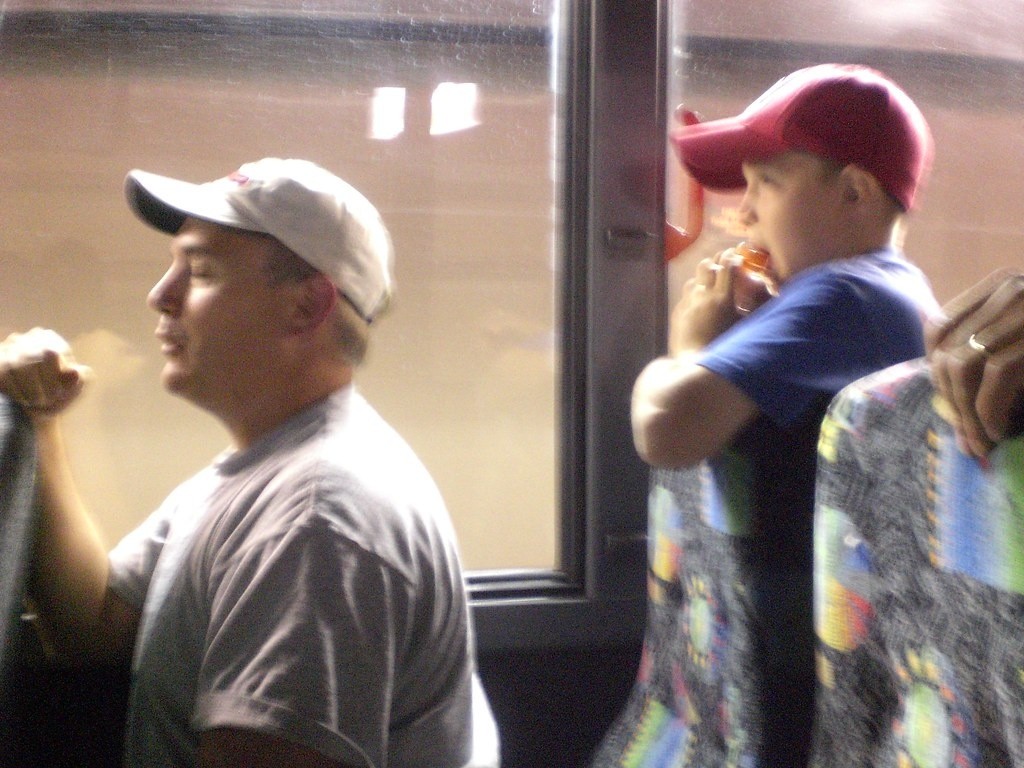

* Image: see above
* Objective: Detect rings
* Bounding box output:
[967,334,992,358]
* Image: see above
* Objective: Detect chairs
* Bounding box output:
[582,354,1024,768]
[0,389,39,768]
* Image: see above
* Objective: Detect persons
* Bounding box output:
[924,269,1024,458]
[0,156,475,768]
[631,62,940,591]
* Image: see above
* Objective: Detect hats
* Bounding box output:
[125,158,391,322]
[670,64,928,213]
